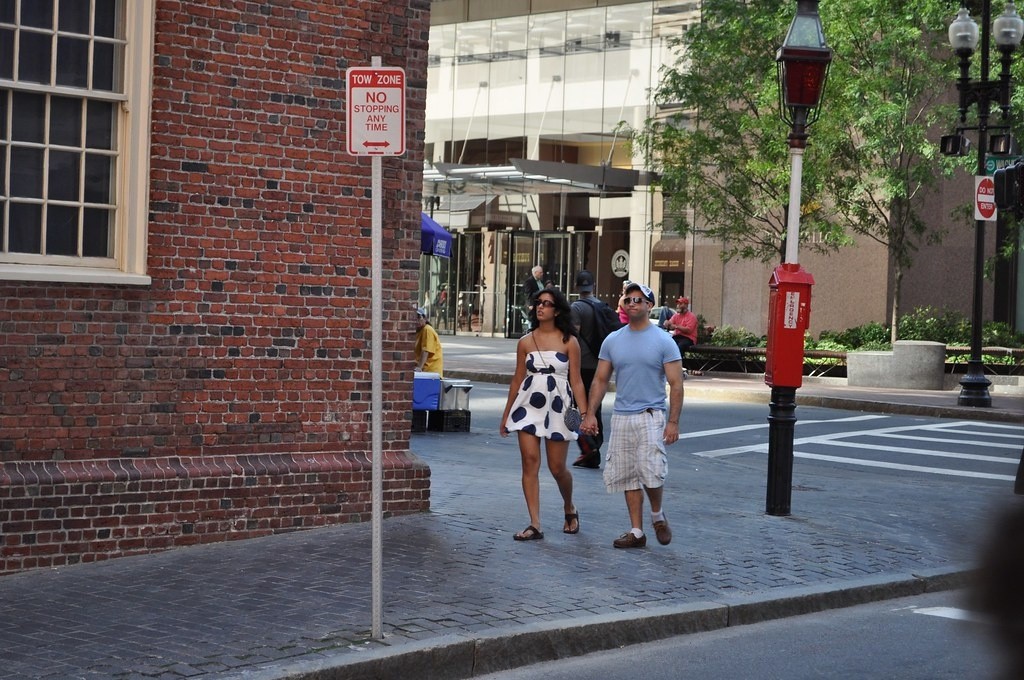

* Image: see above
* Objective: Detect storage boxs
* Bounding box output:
[411,372,471,433]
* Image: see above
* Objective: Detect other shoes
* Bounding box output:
[682,366,687,372]
[572,449,601,469]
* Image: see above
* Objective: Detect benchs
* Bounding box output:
[682,347,847,378]
[946,344,1024,375]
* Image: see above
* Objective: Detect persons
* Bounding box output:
[579,282,684,547]
[568,271,604,467]
[522,266,554,332]
[662,297,698,358]
[413,306,443,380]
[423,283,465,331]
[617,281,634,326]
[500,291,599,540]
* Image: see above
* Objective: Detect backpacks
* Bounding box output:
[575,298,623,360]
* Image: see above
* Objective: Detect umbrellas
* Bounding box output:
[421,211,454,258]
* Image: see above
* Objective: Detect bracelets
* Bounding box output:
[667,419,679,424]
[675,326,678,328]
[580,411,587,417]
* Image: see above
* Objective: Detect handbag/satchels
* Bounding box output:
[565,406,581,432]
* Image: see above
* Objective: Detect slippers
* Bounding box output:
[563,510,579,534]
[513,525,544,541]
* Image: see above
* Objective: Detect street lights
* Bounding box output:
[764,3,834,516]
[940,0,1024,406]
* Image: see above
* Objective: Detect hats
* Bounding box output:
[623,283,655,309]
[576,270,594,293]
[675,298,689,305]
[417,307,424,315]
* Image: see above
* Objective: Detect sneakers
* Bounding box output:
[651,512,672,546]
[613,533,647,548]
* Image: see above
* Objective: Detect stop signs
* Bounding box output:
[974,174,999,221]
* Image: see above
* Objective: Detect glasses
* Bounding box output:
[532,299,555,308]
[624,297,649,305]
